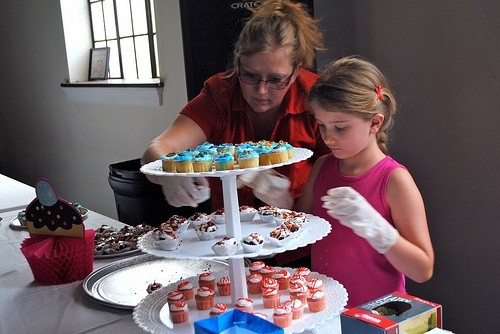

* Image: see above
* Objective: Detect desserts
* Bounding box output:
[152,205,306,256]
[160,140,292,173]
[90,223,153,254]
[166,259,327,329]
[17,201,82,226]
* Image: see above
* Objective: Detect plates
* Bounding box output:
[83,254,251,310]
[93,247,141,259]
[9,207,89,229]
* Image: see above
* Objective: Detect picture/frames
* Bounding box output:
[89,47,110,80]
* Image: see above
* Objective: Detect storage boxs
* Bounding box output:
[340,291,442,334]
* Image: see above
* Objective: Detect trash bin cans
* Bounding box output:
[107,158,184,227]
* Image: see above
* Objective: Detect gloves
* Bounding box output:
[240,170,294,209]
[322,187,399,255]
[162,175,209,207]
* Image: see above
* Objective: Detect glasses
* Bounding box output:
[238,55,296,90]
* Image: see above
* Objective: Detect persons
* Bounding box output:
[141,0,331,274]
[235,56,434,310]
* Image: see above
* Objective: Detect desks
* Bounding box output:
[0,173,350,334]
